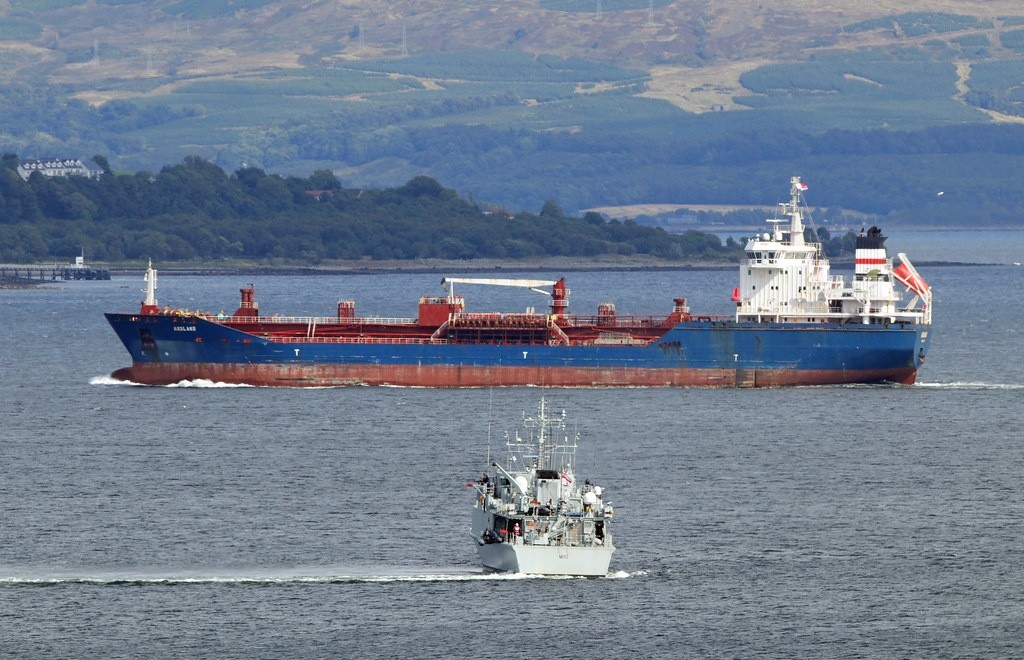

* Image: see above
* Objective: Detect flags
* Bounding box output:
[562,469,572,483]
[796,180,808,190]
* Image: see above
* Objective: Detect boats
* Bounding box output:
[100,175,934,392]
[471,382,618,577]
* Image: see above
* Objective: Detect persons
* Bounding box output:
[513,522,521,540]
[483,472,489,483]
